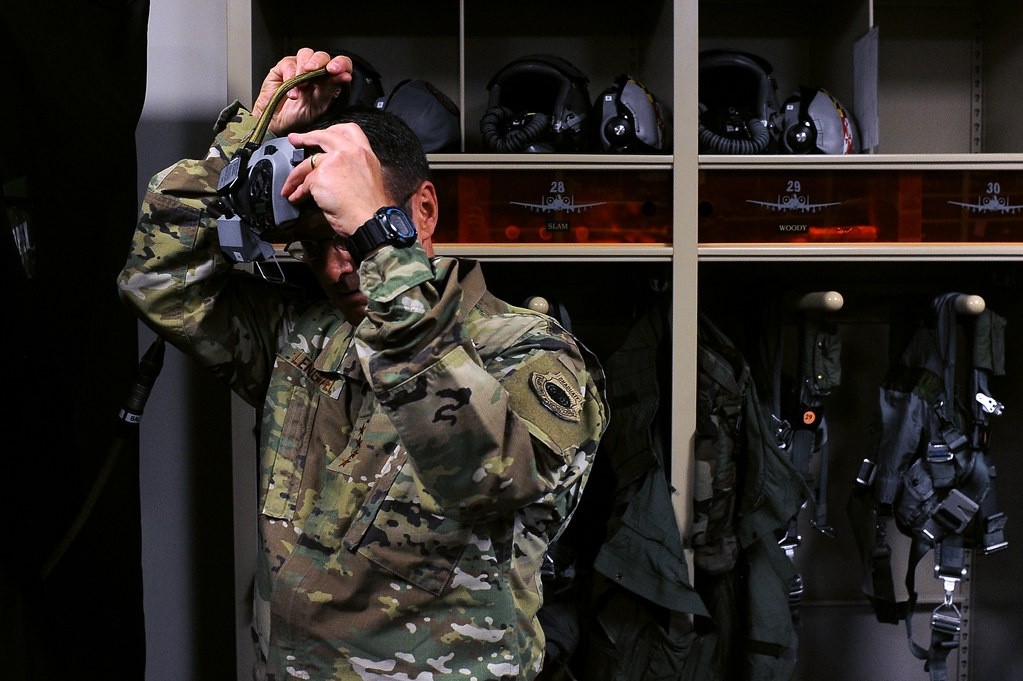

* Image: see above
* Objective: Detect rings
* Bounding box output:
[310,154,316,171]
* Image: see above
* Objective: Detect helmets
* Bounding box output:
[698,49,783,150]
[479,43,592,150]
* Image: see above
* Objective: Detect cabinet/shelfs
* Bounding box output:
[226,0,1023,681]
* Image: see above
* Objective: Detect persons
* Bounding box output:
[118,47,608,680]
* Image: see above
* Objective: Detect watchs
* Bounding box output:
[346,204,419,270]
